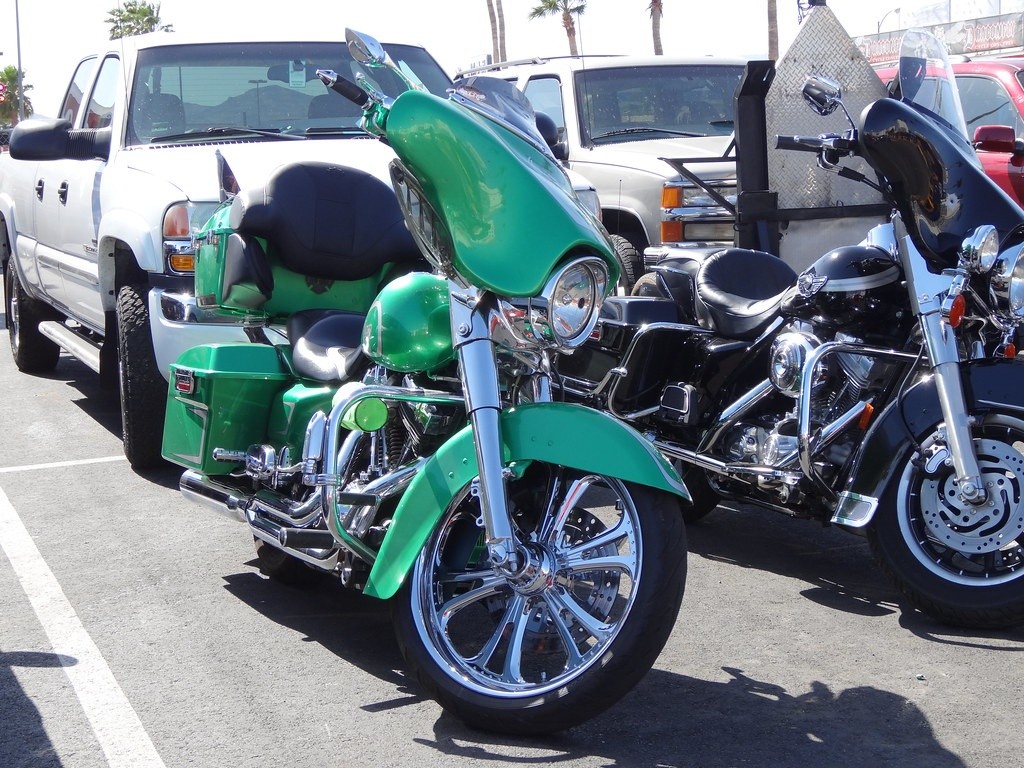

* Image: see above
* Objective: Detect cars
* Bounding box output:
[871,55,1024,210]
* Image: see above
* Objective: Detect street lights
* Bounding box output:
[877,8,901,33]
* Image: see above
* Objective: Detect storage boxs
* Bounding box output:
[558,295,685,409]
[161,343,302,477]
[193,205,234,312]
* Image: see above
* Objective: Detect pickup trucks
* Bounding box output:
[0,26,453,462]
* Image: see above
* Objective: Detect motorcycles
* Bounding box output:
[549,28,1024,629]
[160,23,702,735]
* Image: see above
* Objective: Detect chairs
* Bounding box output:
[143,93,185,139]
[307,94,363,119]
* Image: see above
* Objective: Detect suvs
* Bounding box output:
[455,54,768,288]
